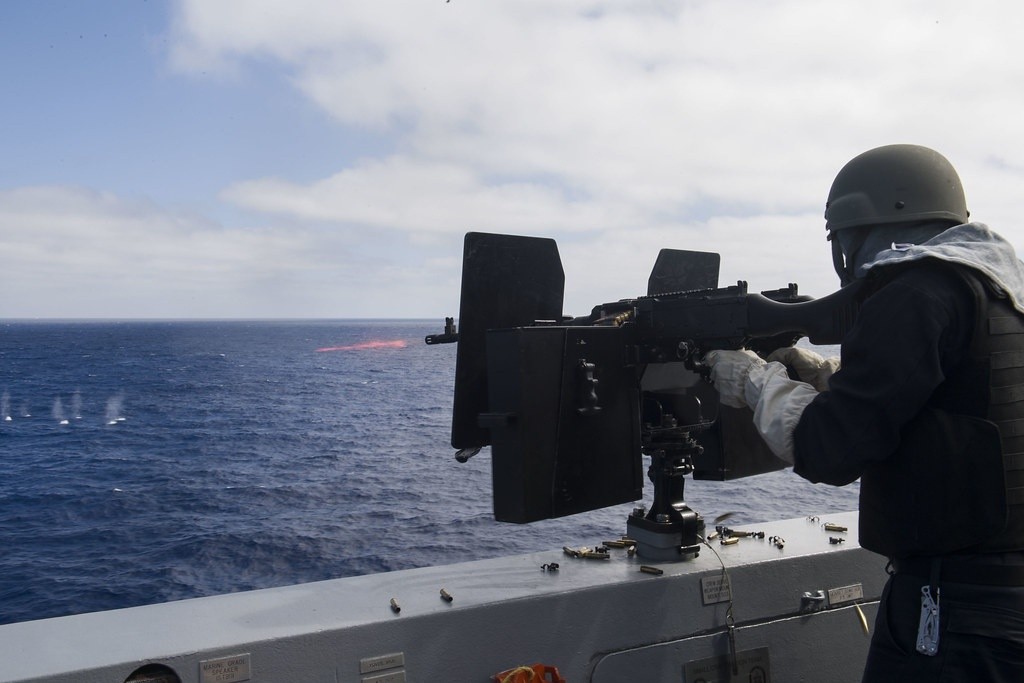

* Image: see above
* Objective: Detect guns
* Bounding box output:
[423,281,871,562]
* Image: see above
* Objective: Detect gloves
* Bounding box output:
[767,346,842,394]
[701,348,818,466]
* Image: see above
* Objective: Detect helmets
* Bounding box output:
[825,144,968,240]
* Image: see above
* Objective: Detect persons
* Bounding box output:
[704,145,1024,683]
